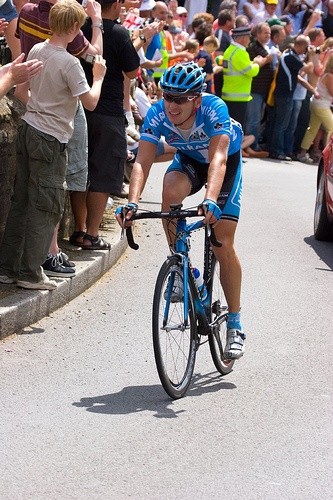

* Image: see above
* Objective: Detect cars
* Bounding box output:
[313,131,333,241]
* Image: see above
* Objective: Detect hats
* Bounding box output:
[265,0,278,4]
[231,27,252,38]
[169,25,181,34]
[267,16,287,26]
[176,7,188,15]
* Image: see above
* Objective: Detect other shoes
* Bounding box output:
[242,144,322,165]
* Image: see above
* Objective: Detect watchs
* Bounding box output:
[92,24,103,29]
[139,34,146,43]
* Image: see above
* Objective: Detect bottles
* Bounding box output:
[191,268,208,301]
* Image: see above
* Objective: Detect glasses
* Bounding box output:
[163,92,198,104]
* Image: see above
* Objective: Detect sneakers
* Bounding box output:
[224,329,246,358]
[0,274,17,284]
[164,275,184,302]
[41,253,76,277]
[16,266,57,290]
[56,251,76,271]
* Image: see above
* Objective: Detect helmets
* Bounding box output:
[160,62,207,92]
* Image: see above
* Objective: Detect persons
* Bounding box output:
[115,60,245,360]
[0,0,333,288]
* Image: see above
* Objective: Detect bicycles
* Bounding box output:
[116,205,235,400]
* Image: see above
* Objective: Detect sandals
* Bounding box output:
[70,231,86,247]
[82,233,111,249]
[126,149,135,162]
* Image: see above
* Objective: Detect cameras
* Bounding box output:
[307,46,320,54]
[85,54,95,64]
